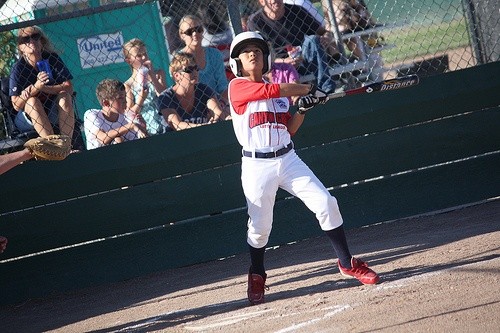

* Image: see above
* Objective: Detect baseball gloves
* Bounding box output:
[24,135,72,160]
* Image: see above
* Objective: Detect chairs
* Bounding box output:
[0,77,86,154]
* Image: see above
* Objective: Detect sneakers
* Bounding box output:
[338,257,380,285]
[247,265,267,304]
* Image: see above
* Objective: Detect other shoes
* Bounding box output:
[322,82,335,94]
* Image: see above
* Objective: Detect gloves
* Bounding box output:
[298,96,318,114]
[304,82,329,104]
[23,134,73,161]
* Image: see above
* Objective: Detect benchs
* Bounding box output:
[295,19,407,93]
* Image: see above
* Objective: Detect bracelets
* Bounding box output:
[135,102,144,108]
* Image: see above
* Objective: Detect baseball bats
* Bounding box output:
[327,75,420,99]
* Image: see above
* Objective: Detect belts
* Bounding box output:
[242,142,295,159]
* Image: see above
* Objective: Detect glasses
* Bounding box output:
[21,33,42,44]
[181,26,204,35]
[178,65,201,72]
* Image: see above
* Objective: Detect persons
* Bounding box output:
[8,25,80,153]
[120,38,169,136]
[227,30,382,304]
[83,79,149,151]
[0,135,72,175]
[157,51,232,132]
[240,1,335,97]
[170,14,232,120]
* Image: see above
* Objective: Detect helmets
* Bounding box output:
[229,32,273,77]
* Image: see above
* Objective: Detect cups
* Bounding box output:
[36,59,53,83]
